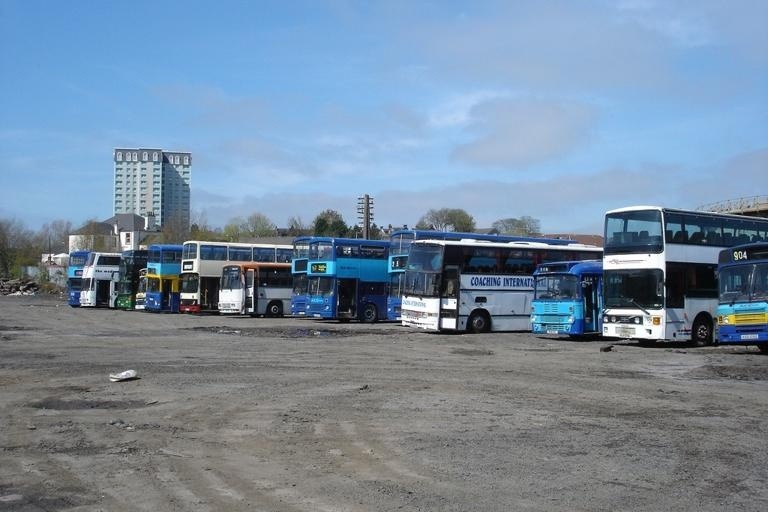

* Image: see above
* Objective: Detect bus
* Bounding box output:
[598,203,767,347]
[712,239,768,357]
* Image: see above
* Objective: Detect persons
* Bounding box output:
[442,280,455,298]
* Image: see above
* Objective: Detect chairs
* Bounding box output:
[638,231,761,252]
[219,249,294,265]
[464,263,532,275]
[336,245,378,258]
